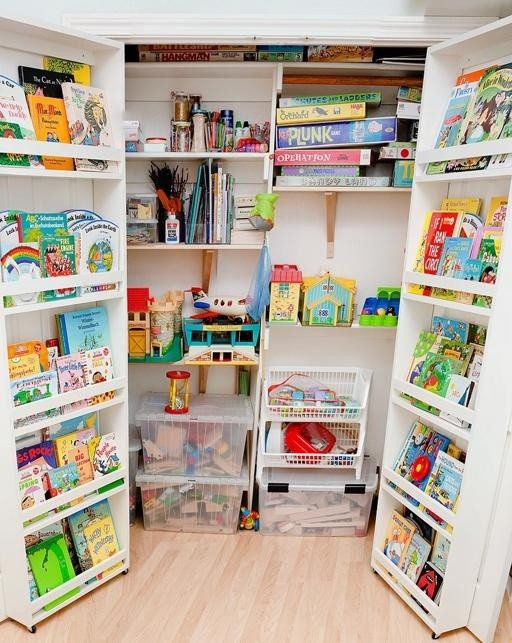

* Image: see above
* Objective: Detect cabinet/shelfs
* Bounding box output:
[123,63,280,533]
[255,62,426,536]
[372,14,512,643]
[0,13,130,634]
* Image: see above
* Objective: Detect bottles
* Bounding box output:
[165,211,180,245]
[169,88,251,152]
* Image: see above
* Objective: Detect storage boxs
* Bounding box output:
[131,392,254,534]
[255,365,379,535]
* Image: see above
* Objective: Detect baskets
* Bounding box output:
[260,421,367,470]
[264,362,372,421]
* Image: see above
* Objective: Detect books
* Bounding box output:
[185,158,235,246]
